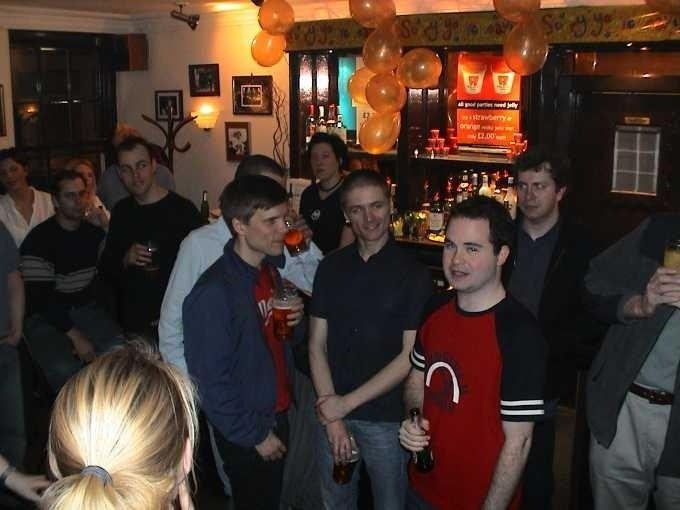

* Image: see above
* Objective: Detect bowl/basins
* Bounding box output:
[318,175,346,193]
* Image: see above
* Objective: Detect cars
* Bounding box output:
[142,241,161,271]
[664,239,680,308]
[284,221,308,258]
[269,281,301,339]
[461,63,484,94]
[490,62,514,95]
[331,448,362,485]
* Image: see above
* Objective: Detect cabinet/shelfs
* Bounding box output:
[305,104,346,146]
[285,183,293,199]
[406,407,435,471]
[201,190,209,219]
[385,170,515,241]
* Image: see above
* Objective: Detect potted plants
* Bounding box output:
[154,90,183,121]
[188,63,221,98]
[232,75,273,115]
[224,121,251,161]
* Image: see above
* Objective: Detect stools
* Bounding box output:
[628,383,674,406]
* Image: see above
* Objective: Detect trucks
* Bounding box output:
[356,114,402,156]
[503,20,550,78]
[363,75,409,116]
[394,47,444,92]
[250,28,287,67]
[361,28,402,74]
[258,0,296,35]
[492,1,543,25]
[346,0,397,31]
[346,65,379,107]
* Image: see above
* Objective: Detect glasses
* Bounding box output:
[169,2,201,32]
[190,109,220,132]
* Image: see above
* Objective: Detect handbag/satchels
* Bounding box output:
[0,465,18,484]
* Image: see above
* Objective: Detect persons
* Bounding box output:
[1,220,31,470]
[0,455,52,504]
[181,172,318,509]
[502,148,597,510]
[297,128,361,252]
[583,206,679,510]
[397,194,553,510]
[156,151,324,414]
[18,166,128,398]
[306,168,434,509]
[37,336,206,509]
[102,132,211,347]
[0,146,59,253]
[65,158,112,227]
